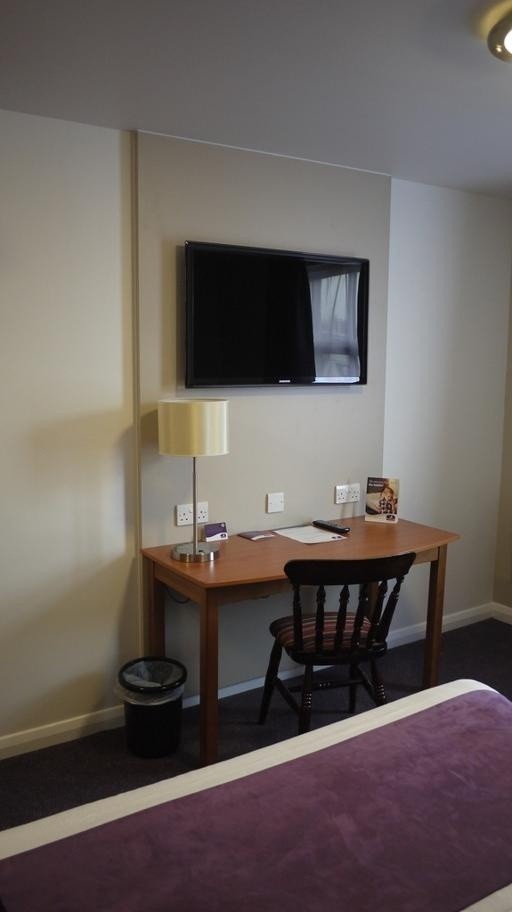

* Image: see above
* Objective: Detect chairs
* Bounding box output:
[255,549,417,736]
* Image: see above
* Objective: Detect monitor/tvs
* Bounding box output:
[183,240,372,389]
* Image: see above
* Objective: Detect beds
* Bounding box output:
[0,673,512,912]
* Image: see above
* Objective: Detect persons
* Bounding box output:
[375,486,398,520]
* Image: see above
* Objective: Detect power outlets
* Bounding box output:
[177,501,208,526]
[334,483,362,504]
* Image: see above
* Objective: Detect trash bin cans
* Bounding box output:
[119,656,187,758]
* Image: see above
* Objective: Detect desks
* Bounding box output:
[132,518,460,770]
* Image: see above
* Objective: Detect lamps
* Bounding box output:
[156,399,231,565]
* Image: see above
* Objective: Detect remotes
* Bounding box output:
[312,519,351,533]
